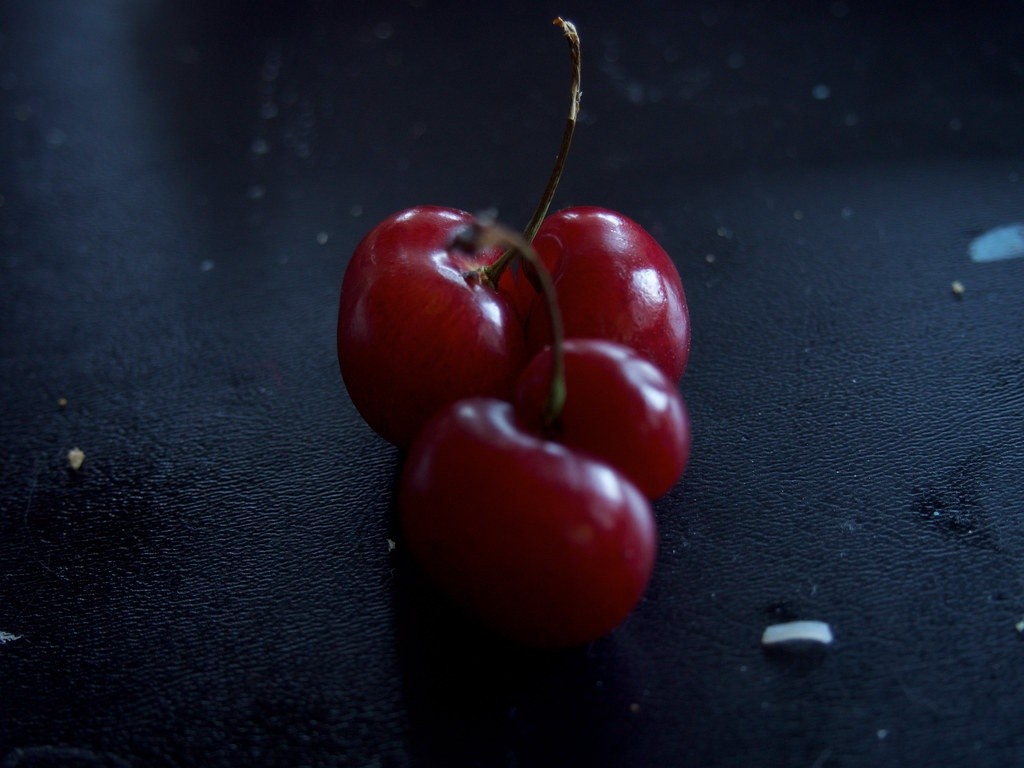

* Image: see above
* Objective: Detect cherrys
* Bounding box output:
[337,206,692,645]
